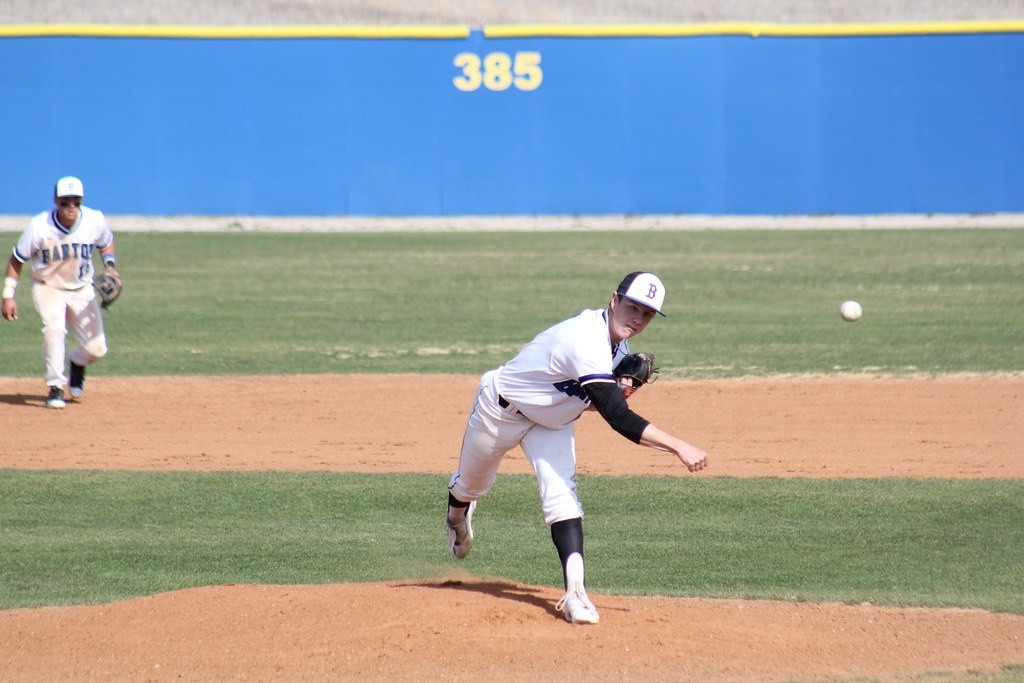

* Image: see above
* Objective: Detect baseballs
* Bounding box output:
[840,299,863,323]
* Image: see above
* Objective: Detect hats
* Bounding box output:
[618,272,668,318]
[55,176,84,198]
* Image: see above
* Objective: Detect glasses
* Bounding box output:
[60,202,81,207]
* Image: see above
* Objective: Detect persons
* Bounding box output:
[2,176,122,409]
[446,272,708,624]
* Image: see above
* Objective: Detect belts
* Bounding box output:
[499,394,523,415]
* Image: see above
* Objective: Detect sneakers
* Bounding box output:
[46,386,66,409]
[68,351,86,398]
[447,499,476,559]
[565,582,600,624]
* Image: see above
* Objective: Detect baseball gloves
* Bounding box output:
[90,264,123,310]
[612,351,655,400]
